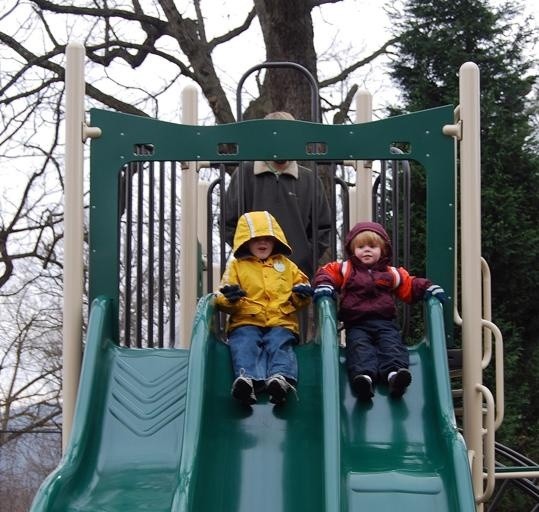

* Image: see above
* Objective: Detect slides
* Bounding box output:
[32,39,503,512]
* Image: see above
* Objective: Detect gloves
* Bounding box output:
[312,284,334,300]
[424,284,449,303]
[220,283,247,303]
[292,283,313,294]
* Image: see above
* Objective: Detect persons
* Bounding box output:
[217,112,333,280]
[218,210,315,406]
[311,222,448,398]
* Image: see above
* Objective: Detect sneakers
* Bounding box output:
[230,376,259,407]
[387,368,412,400]
[351,374,376,403]
[268,375,289,405]
